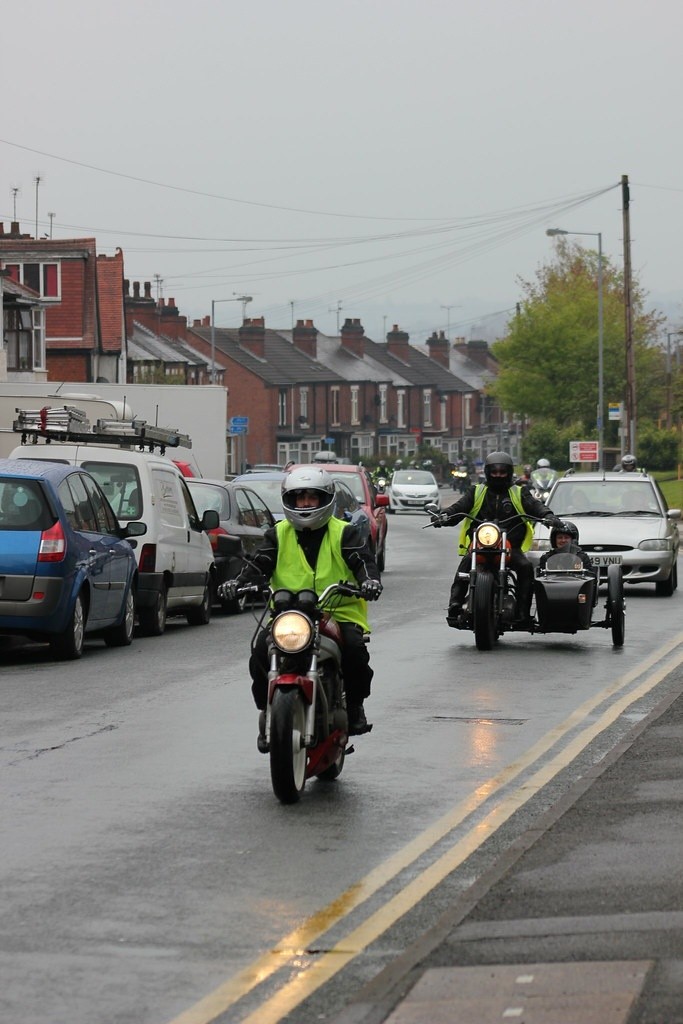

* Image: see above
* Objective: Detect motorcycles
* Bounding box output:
[423,503,627,653]
[519,465,576,503]
[450,466,473,495]
[370,473,394,495]
[214,532,383,805]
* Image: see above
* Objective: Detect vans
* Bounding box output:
[7,395,221,637]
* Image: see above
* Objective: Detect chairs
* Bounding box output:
[620,491,649,509]
[18,500,40,522]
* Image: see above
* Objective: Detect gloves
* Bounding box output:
[356,580,383,602]
[543,514,563,528]
[217,580,242,599]
[432,513,445,528]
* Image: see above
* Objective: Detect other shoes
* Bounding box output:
[343,698,367,734]
[515,609,532,624]
[447,603,463,617]
[256,712,271,752]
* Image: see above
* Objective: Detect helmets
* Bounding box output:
[379,460,386,466]
[537,459,550,467]
[622,454,636,472]
[458,460,464,465]
[409,460,416,466]
[524,465,533,477]
[280,466,336,531]
[426,460,432,465]
[396,459,403,465]
[484,451,514,485]
[550,520,578,549]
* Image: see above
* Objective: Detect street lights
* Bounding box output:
[546,228,606,473]
[211,295,254,385]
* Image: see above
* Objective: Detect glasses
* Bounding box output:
[490,469,509,475]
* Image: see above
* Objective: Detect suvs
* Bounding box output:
[283,461,390,575]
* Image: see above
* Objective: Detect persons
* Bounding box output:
[215,467,384,754]
[431,451,560,621]
[612,455,645,473]
[370,459,432,488]
[455,459,558,487]
[537,521,593,578]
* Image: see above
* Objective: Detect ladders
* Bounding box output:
[13,405,90,433]
[93,417,193,450]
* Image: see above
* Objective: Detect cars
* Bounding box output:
[1,458,148,661]
[529,467,682,598]
[388,470,443,515]
[229,472,289,524]
[183,476,284,614]
